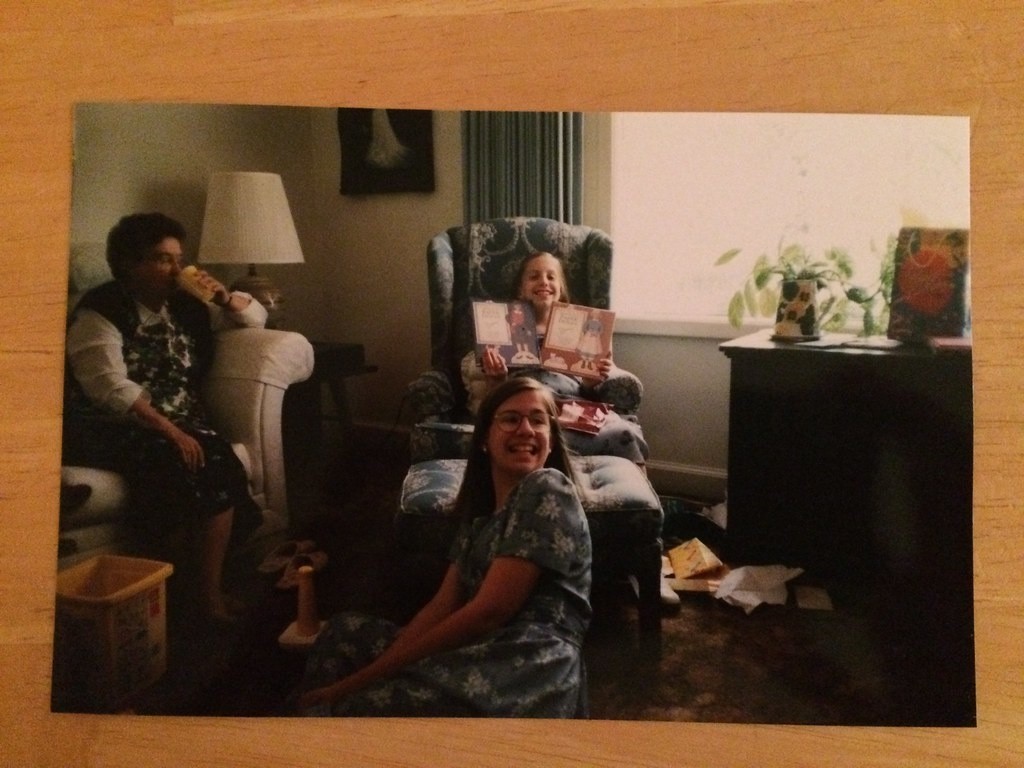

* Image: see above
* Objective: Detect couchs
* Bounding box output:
[56,242,315,573]
[404,215,684,613]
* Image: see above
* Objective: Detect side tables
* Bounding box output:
[279,340,379,472]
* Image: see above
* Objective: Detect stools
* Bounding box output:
[394,454,665,635]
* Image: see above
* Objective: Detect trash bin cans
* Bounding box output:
[56,553,176,709]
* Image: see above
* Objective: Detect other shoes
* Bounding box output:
[181,588,194,613]
[187,609,241,631]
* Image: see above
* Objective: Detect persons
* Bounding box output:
[288,378,592,720]
[575,310,604,372]
[506,300,534,359]
[479,252,680,615]
[60,213,270,625]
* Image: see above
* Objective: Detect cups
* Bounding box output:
[175,265,216,304]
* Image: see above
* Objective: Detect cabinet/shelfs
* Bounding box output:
[718,328,974,591]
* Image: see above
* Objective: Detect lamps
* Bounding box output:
[198,171,305,312]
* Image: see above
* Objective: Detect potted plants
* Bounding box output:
[712,215,905,337]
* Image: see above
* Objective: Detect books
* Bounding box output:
[469,296,616,437]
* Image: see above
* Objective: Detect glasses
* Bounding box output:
[492,410,551,433]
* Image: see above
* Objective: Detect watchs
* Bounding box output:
[223,291,232,305]
